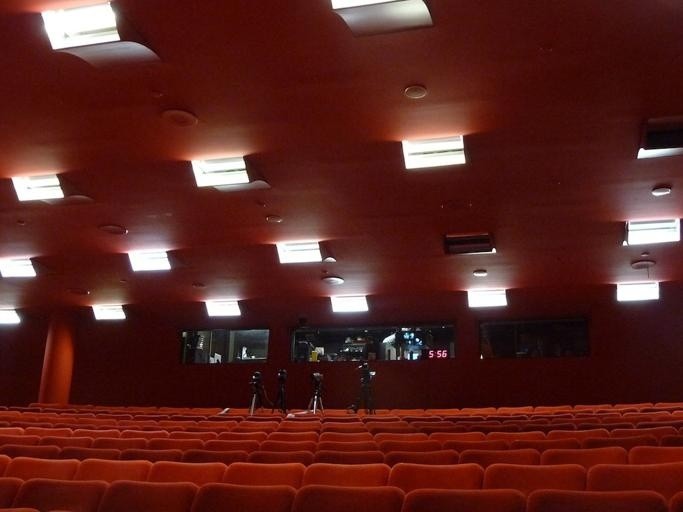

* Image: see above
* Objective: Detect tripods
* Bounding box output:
[247,391,262,415]
[272,386,287,414]
[307,394,324,415]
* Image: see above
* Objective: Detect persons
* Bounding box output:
[308,341,332,362]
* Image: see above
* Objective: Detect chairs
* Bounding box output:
[0,402,683,512]
